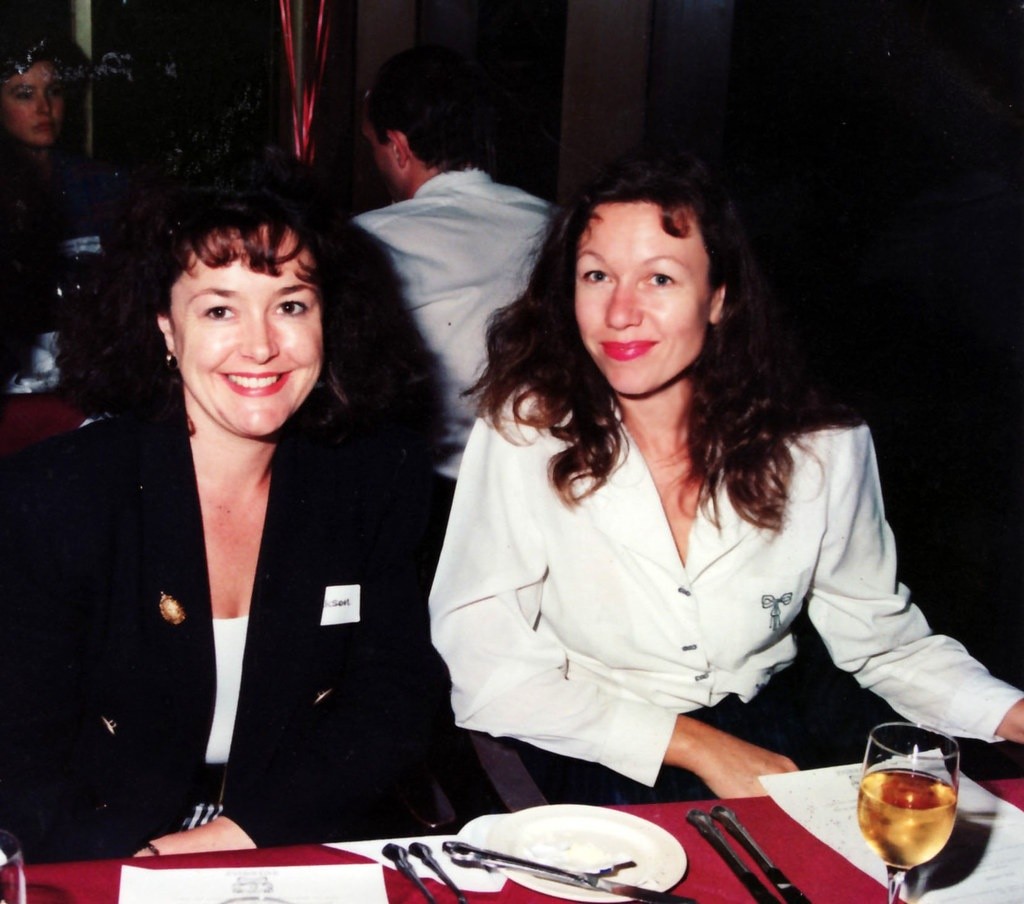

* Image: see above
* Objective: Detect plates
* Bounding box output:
[488,804,688,903]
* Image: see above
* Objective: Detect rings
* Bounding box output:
[148,843,159,855]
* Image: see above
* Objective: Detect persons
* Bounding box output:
[0,199,443,861]
[0,38,578,556]
[426,150,1024,801]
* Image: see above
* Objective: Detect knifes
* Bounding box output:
[710,805,813,904]
[686,809,782,904]
[442,841,702,904]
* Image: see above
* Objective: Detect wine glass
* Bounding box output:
[856,721,959,904]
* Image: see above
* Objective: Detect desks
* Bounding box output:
[0,779,1024,904]
